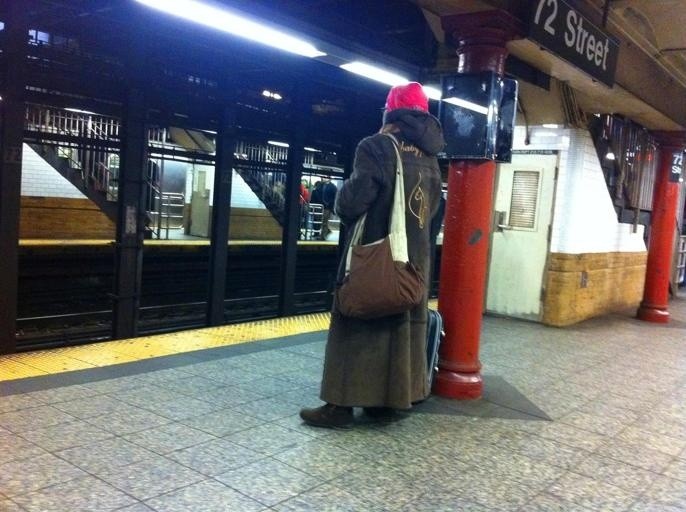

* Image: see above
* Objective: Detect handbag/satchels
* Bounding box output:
[333,235,423,318]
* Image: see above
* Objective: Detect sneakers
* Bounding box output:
[361,406,389,417]
[300,403,353,430]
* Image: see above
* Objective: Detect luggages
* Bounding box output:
[424,308,445,398]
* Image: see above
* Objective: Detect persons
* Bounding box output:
[315,174,338,244]
[145,159,161,214]
[298,183,311,240]
[299,80,448,431]
[309,181,321,238]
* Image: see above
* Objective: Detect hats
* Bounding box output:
[387,82,429,111]
